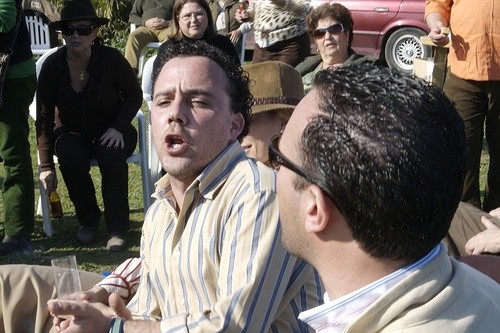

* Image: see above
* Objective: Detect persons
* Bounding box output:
[440,201,500,256]
[235,0,315,67]
[0,0,40,257]
[268,63,500,333]
[47,37,322,333]
[123,0,311,170]
[294,2,367,97]
[423,0,500,213]
[36,0,143,254]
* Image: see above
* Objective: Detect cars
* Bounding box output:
[222,0,437,78]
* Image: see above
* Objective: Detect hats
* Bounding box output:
[238,60,305,114]
[48,0,110,30]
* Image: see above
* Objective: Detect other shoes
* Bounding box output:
[0,225,34,255]
[76,222,101,244]
[106,231,131,252]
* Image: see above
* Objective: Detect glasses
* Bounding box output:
[267,133,343,215]
[313,23,345,38]
[61,24,94,36]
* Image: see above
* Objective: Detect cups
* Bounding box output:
[51,255,84,320]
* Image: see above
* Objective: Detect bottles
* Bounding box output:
[50,190,63,219]
[239,0,249,22]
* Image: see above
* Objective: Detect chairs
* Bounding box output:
[25,16,51,54]
[142,55,162,189]
[130,23,162,78]
[30,47,152,236]
[216,13,256,63]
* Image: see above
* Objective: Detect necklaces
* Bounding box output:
[69,61,88,80]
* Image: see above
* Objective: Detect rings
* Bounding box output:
[427,35,431,38]
